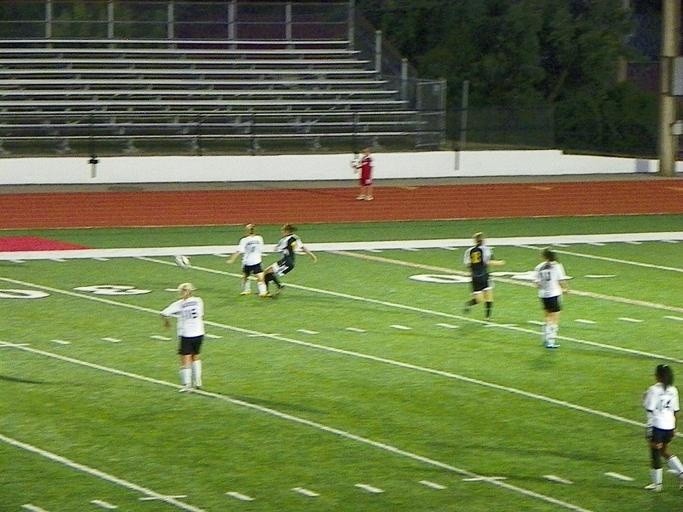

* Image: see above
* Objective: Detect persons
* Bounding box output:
[639,363,683,491]
[159,282,205,393]
[533,247,569,348]
[352,147,374,201]
[226,223,273,298]
[262,224,317,298]
[460,232,506,324]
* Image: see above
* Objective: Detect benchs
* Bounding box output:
[0,34,440,150]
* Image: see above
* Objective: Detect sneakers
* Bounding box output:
[644,483,663,493]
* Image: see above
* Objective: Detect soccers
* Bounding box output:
[175,256,190,268]
